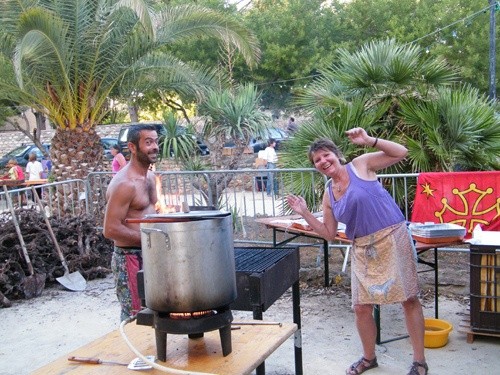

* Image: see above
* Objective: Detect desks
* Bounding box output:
[254,208,465,345]
[34,320,299,375]
[0,178,49,205]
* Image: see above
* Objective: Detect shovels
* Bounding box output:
[68,354,154,370]
[3,184,46,299]
[32,185,86,292]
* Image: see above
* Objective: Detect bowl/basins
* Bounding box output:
[423,317,453,348]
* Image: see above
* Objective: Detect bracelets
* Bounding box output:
[372,138,378,148]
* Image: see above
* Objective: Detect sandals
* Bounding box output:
[345,356,378,375]
[407,361,428,375]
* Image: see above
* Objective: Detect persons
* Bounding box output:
[287,126,429,375]
[264,139,280,200]
[103,122,164,324]
[286,117,299,137]
[24,152,44,202]
[0,159,27,207]
[252,136,268,191]
[40,152,52,201]
[109,144,127,178]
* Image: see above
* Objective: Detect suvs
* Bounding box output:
[0,143,51,168]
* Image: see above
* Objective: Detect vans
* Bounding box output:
[119,124,191,162]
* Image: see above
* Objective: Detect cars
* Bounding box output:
[195,128,296,155]
[99,138,122,159]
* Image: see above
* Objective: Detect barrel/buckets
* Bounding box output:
[139,201,237,313]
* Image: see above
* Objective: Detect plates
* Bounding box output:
[406,223,467,243]
